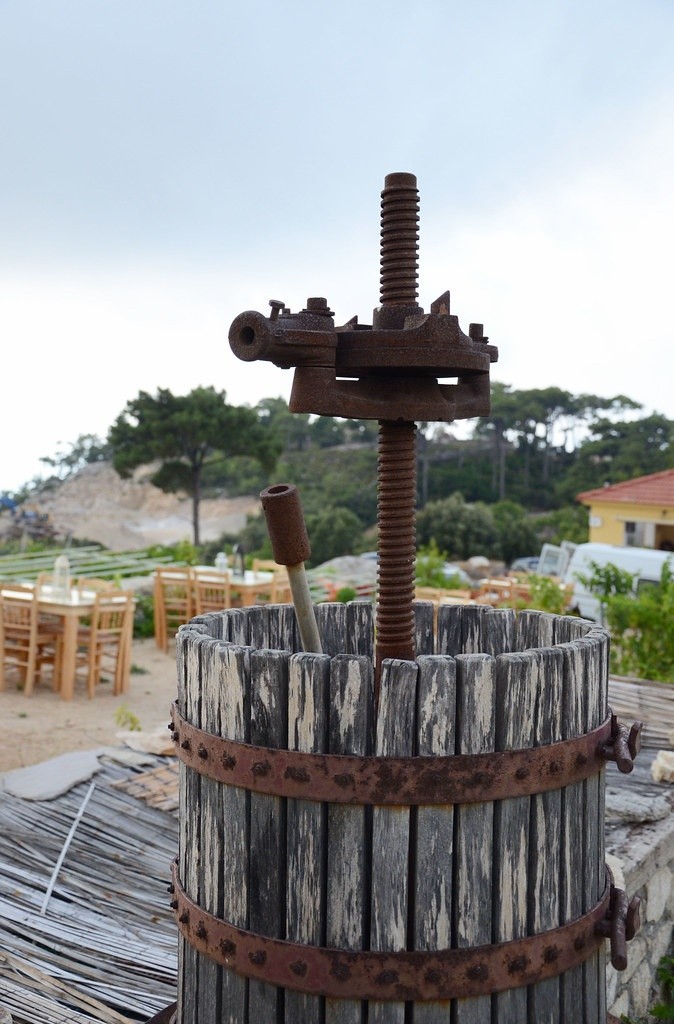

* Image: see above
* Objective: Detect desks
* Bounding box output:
[0,579,139,698]
[151,563,296,651]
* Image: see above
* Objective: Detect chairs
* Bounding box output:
[411,567,576,638]
[155,566,197,654]
[194,568,241,616]
[68,589,132,698]
[29,572,71,683]
[67,577,118,687]
[0,583,60,693]
[223,553,245,608]
[262,564,295,605]
[252,557,279,606]
